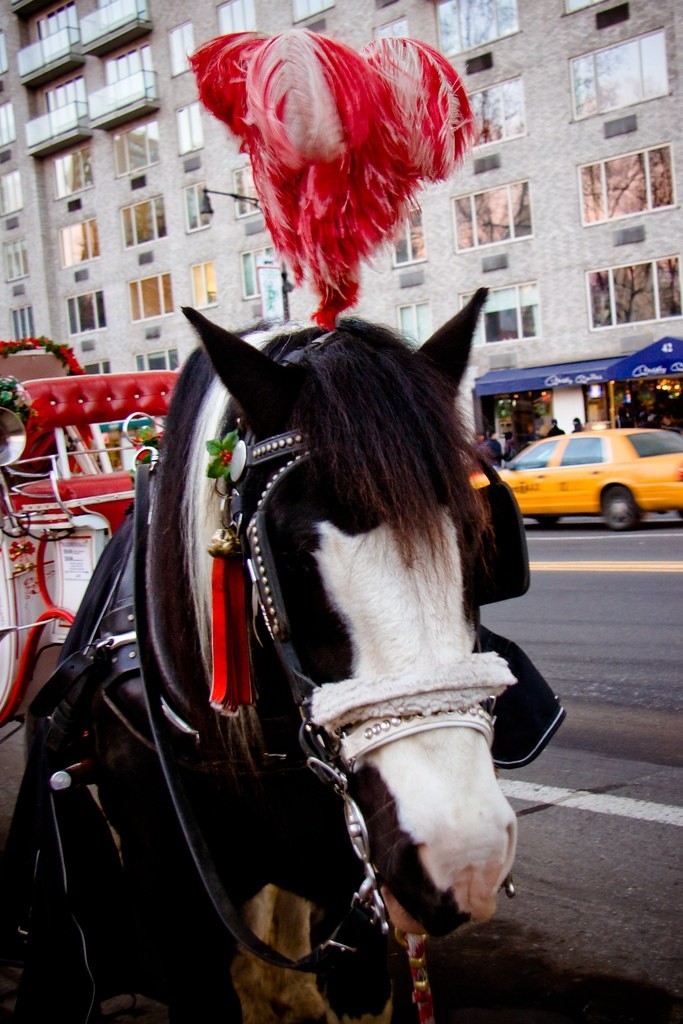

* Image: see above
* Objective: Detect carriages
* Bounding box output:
[0,24,571,1024]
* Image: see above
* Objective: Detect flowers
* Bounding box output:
[0,375,38,426]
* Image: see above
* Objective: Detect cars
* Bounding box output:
[492,425,683,532]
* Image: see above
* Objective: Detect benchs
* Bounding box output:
[0,368,180,530]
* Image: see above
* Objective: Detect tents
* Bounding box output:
[607,337,683,428]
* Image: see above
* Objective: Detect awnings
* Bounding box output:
[475,357,628,397]
[100,416,155,432]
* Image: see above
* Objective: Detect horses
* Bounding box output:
[0,287,518,1024]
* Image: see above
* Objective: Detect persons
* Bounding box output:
[572,418,584,432]
[475,430,504,471]
[615,407,661,429]
[503,432,520,461]
[547,419,565,436]
